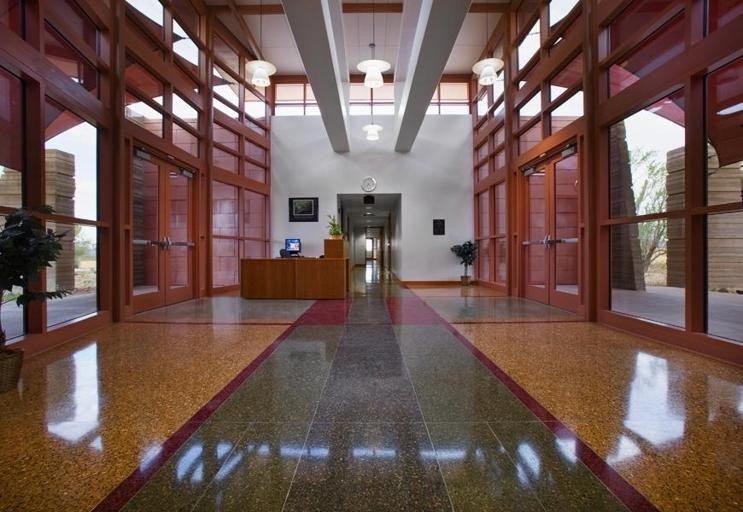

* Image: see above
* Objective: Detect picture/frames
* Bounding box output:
[289,197,319,223]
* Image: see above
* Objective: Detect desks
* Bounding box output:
[240,256,350,301]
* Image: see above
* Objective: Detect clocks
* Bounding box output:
[360,175,377,192]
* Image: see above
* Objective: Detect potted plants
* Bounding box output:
[0,204,74,392]
[451,240,477,286]
[325,213,344,240]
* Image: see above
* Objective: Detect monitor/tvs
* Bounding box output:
[285,239,301,252]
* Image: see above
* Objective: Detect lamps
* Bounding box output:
[472,1,506,87]
[355,0,391,90]
[243,1,276,88]
[361,88,382,140]
